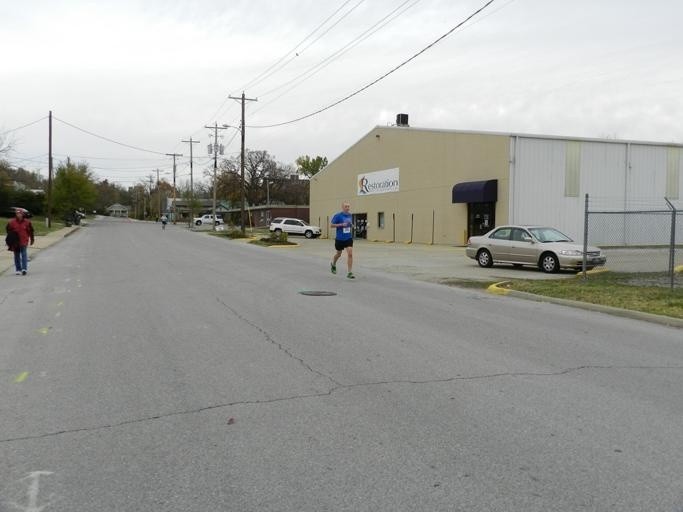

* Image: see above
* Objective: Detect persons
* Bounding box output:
[71,208,76,223]
[5,208,34,276]
[159,213,167,232]
[328,199,356,279]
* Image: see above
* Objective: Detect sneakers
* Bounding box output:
[330,260,337,274]
[346,271,356,279]
[14,268,28,276]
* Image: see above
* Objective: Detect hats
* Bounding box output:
[15,207,25,216]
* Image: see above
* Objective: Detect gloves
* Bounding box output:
[29,233,36,246]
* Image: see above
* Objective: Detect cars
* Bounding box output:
[10,206,32,218]
[193,214,224,226]
[269,216,322,239]
[465,225,607,274]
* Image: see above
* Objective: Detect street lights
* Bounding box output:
[223,124,245,233]
[266,181,275,205]
